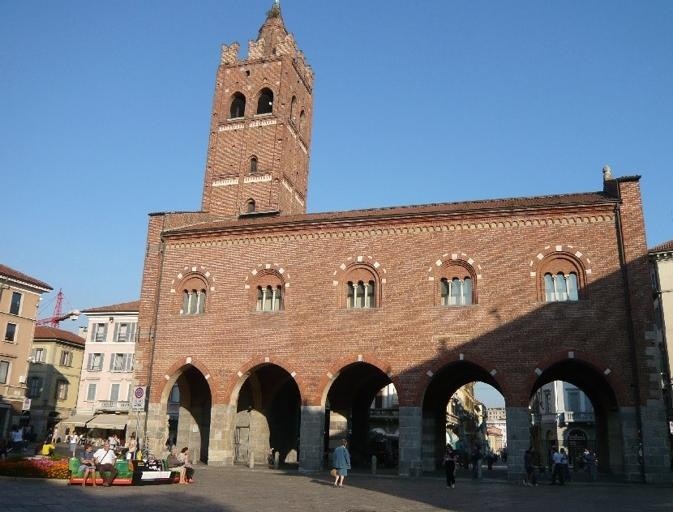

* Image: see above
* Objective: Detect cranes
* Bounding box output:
[35,287,80,328]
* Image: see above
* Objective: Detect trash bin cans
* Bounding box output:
[409,460,424,477]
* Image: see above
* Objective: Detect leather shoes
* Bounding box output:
[178,477,195,485]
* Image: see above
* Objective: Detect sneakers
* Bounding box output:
[445,483,456,490]
[81,481,111,489]
[522,478,592,487]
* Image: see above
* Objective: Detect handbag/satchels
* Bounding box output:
[94,462,102,471]
[329,468,337,477]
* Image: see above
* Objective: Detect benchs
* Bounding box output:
[67,456,182,488]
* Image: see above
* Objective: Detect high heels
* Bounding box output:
[332,484,344,488]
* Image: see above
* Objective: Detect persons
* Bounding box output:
[518,445,596,488]
[458,447,496,480]
[442,444,456,489]
[330,438,351,487]
[0,421,195,487]
